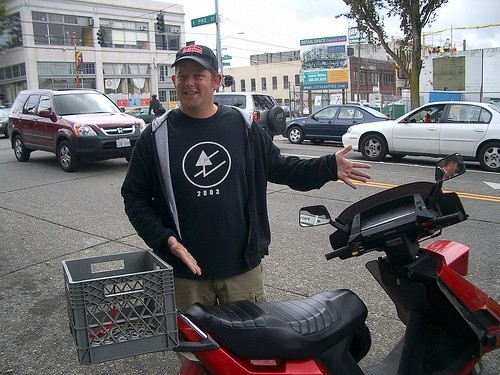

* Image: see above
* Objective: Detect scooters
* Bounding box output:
[173,151,500,375]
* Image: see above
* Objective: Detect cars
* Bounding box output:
[280,103,392,145]
[0,110,11,139]
[124,106,158,125]
[341,100,500,173]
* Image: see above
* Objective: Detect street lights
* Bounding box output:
[216,31,245,93]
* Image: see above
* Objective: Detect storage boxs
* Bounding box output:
[63,249,180,365]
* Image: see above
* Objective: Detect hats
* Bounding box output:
[444,155,463,164]
[171,45,219,73]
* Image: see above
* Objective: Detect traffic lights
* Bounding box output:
[76,51,84,67]
[156,14,166,33]
[96,30,105,45]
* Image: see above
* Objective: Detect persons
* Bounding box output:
[429,38,457,54]
[121,45,371,364]
[149,94,162,115]
[440,154,462,181]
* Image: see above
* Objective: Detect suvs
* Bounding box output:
[9,88,147,173]
[213,90,287,143]
